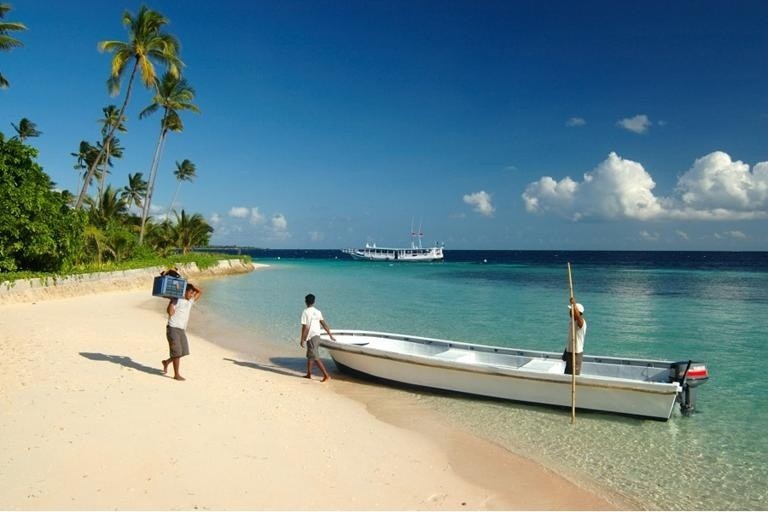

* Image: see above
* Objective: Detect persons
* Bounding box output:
[162,282,202,380]
[561,297,586,375]
[299,294,336,382]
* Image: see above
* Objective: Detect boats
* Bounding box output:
[319,328,709,421]
[350,218,444,261]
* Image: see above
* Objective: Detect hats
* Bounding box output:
[567,302,585,315]
